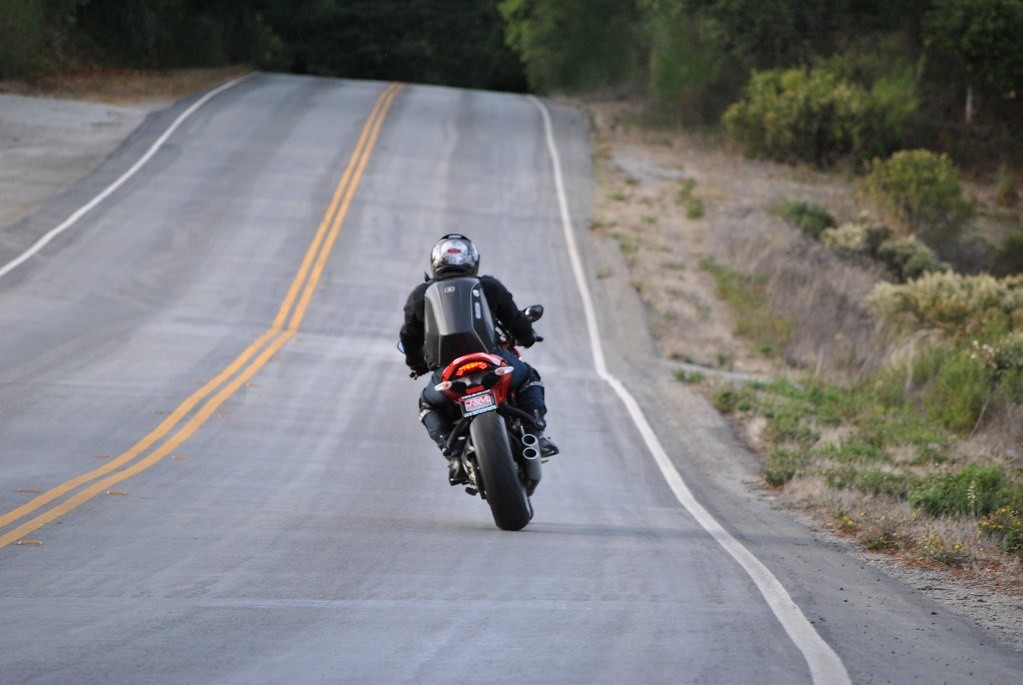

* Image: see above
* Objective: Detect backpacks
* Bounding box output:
[422,277,496,372]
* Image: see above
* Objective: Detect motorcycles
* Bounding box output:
[395,303,564,532]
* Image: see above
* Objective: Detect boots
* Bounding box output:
[529,407,559,457]
[432,428,461,485]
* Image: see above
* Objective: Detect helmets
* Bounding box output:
[430,233,480,276]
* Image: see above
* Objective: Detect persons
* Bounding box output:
[400,234,559,486]
